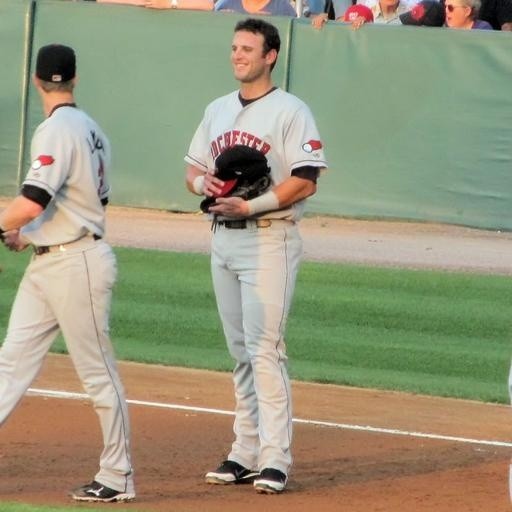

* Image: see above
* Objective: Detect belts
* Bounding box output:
[33,234,99,256]
[217,219,274,229]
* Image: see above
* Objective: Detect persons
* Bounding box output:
[76,0,512,33]
[185,17,331,492]
[0,42,139,503]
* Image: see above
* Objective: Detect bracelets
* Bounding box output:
[190,171,208,196]
[244,191,283,218]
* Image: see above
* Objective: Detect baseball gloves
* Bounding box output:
[230,175,271,199]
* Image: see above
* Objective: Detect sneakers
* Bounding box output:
[66,461,288,503]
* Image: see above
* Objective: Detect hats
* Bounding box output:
[343,0,445,26]
[211,145,268,198]
[36,44,76,84]
